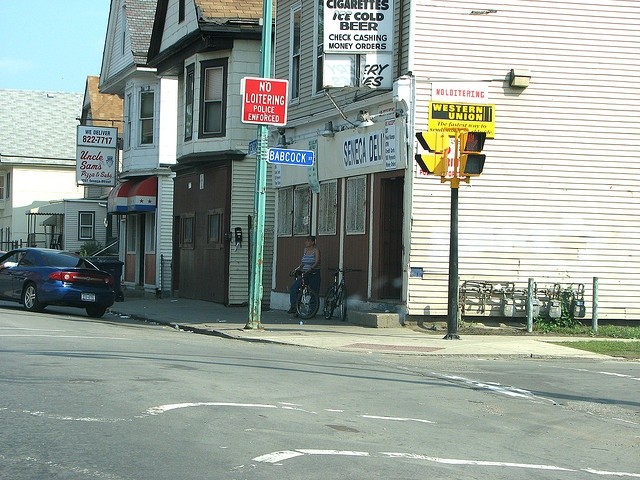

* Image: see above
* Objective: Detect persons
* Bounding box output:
[0,253,25,270]
[287,236,321,318]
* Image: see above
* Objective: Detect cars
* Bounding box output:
[0,251,19,269]
[0,247,116,318]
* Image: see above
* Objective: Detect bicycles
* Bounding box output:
[323,267,362,321]
[290,269,320,319]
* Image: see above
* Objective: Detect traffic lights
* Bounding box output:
[415,153,449,175]
[416,131,450,152]
[459,131,487,154]
[459,152,486,176]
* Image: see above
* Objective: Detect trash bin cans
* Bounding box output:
[91,256,125,302]
